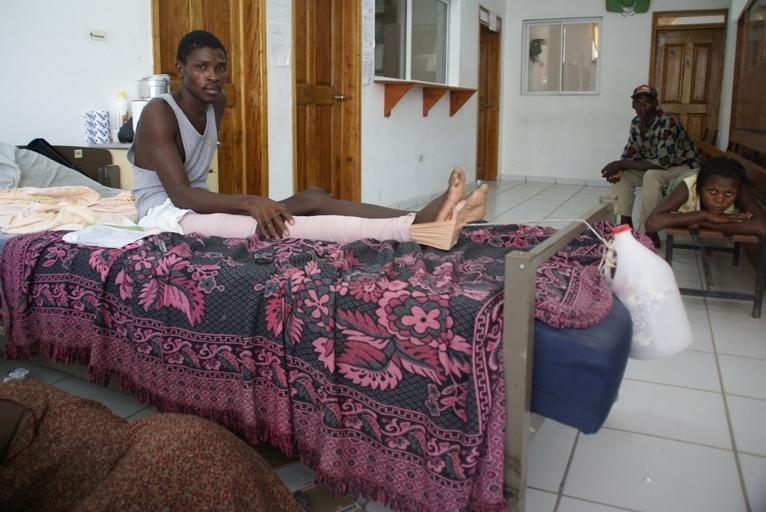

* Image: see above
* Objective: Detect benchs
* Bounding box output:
[666,130,766,319]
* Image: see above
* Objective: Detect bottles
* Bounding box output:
[116,89,134,143]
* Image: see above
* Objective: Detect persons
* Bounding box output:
[644,158,766,239]
[600,85,699,245]
[126,30,491,246]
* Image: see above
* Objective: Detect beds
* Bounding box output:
[0,146,633,512]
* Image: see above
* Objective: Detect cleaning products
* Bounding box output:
[116,89,128,126]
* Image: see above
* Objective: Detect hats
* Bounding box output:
[631,84,657,99]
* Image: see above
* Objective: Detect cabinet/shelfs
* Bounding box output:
[92,142,221,195]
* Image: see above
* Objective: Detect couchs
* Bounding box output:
[0,379,300,512]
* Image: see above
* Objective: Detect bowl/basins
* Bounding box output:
[138,79,168,99]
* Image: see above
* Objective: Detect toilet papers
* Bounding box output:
[131,99,149,134]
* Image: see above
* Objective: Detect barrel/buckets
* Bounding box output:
[602,222,695,360]
